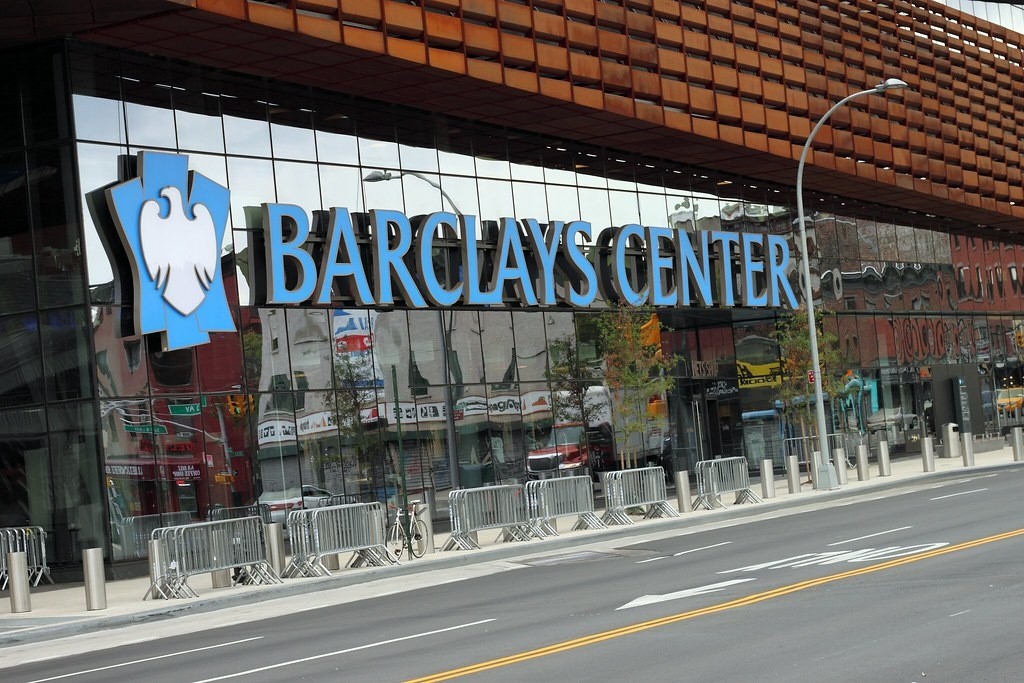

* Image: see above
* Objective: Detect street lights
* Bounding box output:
[794,77,909,489]
[367,170,473,487]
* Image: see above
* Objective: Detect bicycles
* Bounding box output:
[384,499,429,564]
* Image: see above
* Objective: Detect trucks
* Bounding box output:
[523,388,669,490]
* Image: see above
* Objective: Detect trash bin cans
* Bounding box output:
[941,422,960,458]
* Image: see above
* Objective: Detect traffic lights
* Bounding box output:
[1016,332,1024,350]
[226,393,256,418]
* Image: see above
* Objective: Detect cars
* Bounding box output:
[996,386,1024,418]
[252,484,333,540]
[866,407,920,434]
